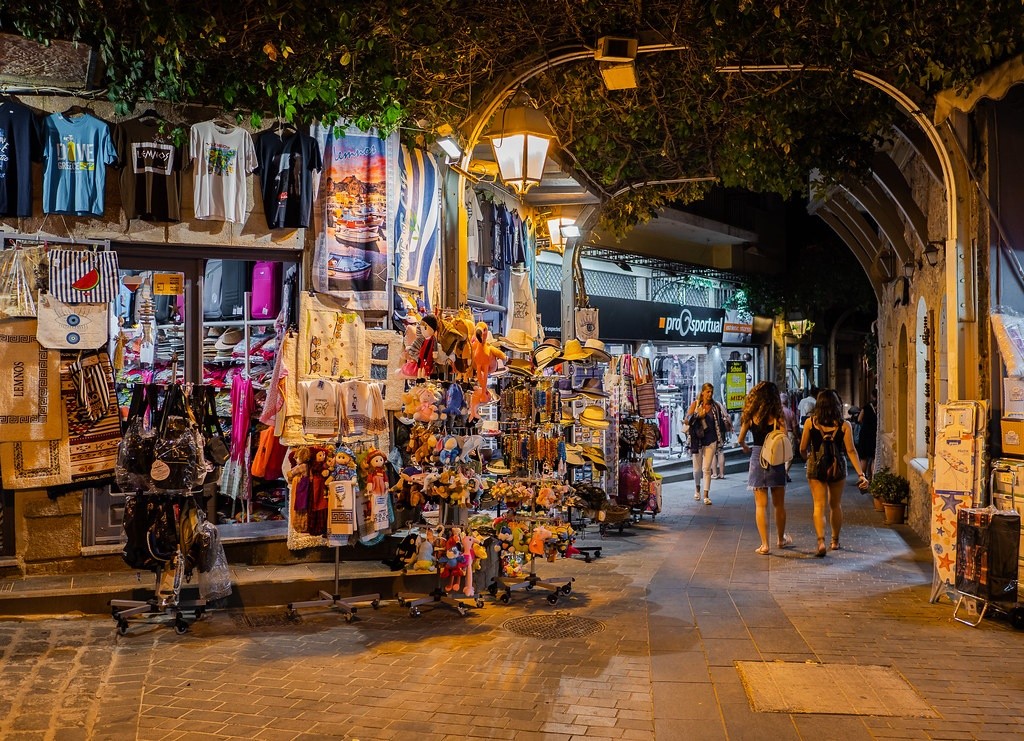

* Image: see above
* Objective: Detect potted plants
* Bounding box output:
[871,466,910,525]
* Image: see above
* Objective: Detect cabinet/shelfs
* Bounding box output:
[106,358,206,636]
[108,283,290,522]
[286,372,380,624]
[394,304,658,618]
[654,378,696,460]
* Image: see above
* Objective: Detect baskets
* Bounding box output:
[598,505,630,524]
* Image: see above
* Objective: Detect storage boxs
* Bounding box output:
[992,378,1024,609]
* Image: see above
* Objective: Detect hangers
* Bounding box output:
[466,176,533,225]
[0,83,299,135]
[655,352,694,360]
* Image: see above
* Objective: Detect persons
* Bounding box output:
[779,387,877,482]
[738,382,793,554]
[286,445,389,537]
[681,382,732,505]
[799,389,869,556]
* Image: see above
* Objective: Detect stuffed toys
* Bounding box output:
[396,528,487,597]
[490,481,576,510]
[386,317,507,508]
[494,524,579,561]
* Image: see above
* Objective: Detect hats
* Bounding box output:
[496,329,612,470]
[202,325,242,362]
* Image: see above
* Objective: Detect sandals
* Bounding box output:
[831,535,839,550]
[815,536,827,558]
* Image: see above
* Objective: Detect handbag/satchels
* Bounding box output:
[607,355,668,514]
[116,384,229,583]
[1,250,118,486]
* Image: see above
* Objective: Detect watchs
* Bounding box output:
[859,473,865,477]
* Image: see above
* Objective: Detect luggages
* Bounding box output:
[952,467,1024,630]
[117,257,280,324]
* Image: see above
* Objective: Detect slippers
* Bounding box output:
[755,544,772,555]
[778,534,792,549]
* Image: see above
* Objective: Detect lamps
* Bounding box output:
[615,262,633,272]
[483,88,558,204]
[436,136,466,162]
[546,215,577,252]
[787,304,807,339]
[923,237,946,267]
[729,282,743,289]
[902,257,923,277]
[662,270,677,277]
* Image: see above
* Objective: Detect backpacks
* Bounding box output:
[760,417,793,469]
[810,416,843,482]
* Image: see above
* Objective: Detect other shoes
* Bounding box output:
[704,498,712,505]
[694,492,701,501]
